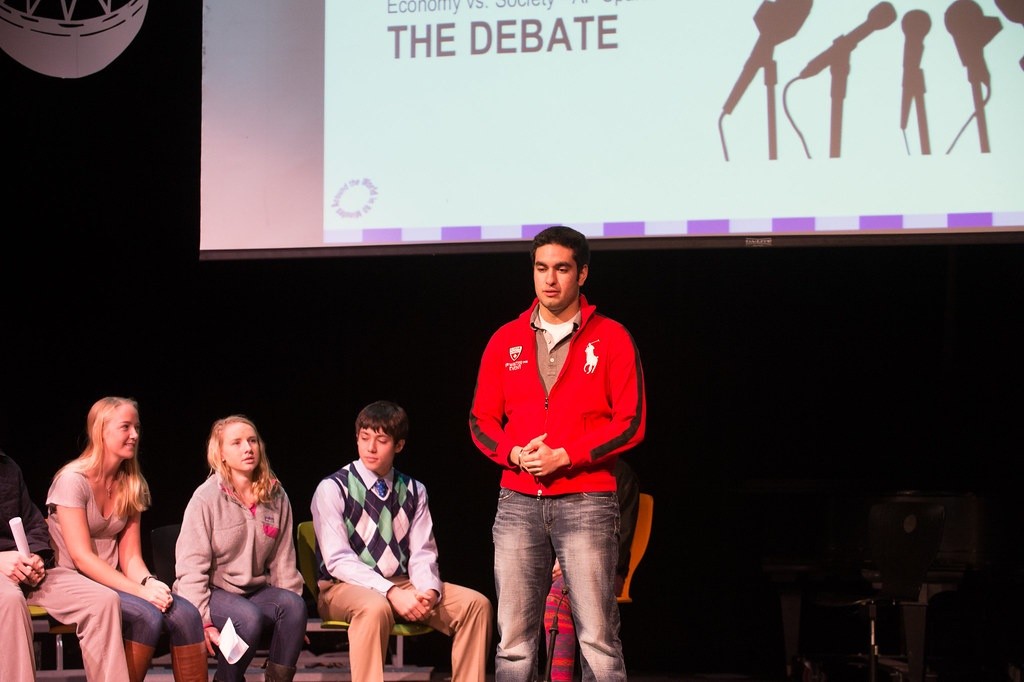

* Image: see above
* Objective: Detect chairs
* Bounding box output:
[614,493,653,601]
[790,490,954,682]
[28,521,435,682]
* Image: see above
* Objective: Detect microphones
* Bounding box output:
[543,586,570,682]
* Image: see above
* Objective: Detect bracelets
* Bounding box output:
[518,446,528,468]
[141,573,158,588]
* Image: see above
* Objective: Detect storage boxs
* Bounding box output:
[937,494,988,569]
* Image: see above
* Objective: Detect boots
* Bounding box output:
[124,639,154,682]
[170,641,210,682]
[264,662,297,682]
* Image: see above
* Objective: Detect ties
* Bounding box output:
[375,479,388,497]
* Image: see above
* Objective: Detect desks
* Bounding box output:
[764,558,985,682]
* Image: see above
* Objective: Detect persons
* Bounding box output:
[542,451,641,682]
[307,401,495,681]
[170,415,312,682]
[466,222,647,681]
[49,395,214,682]
[0,446,133,682]
